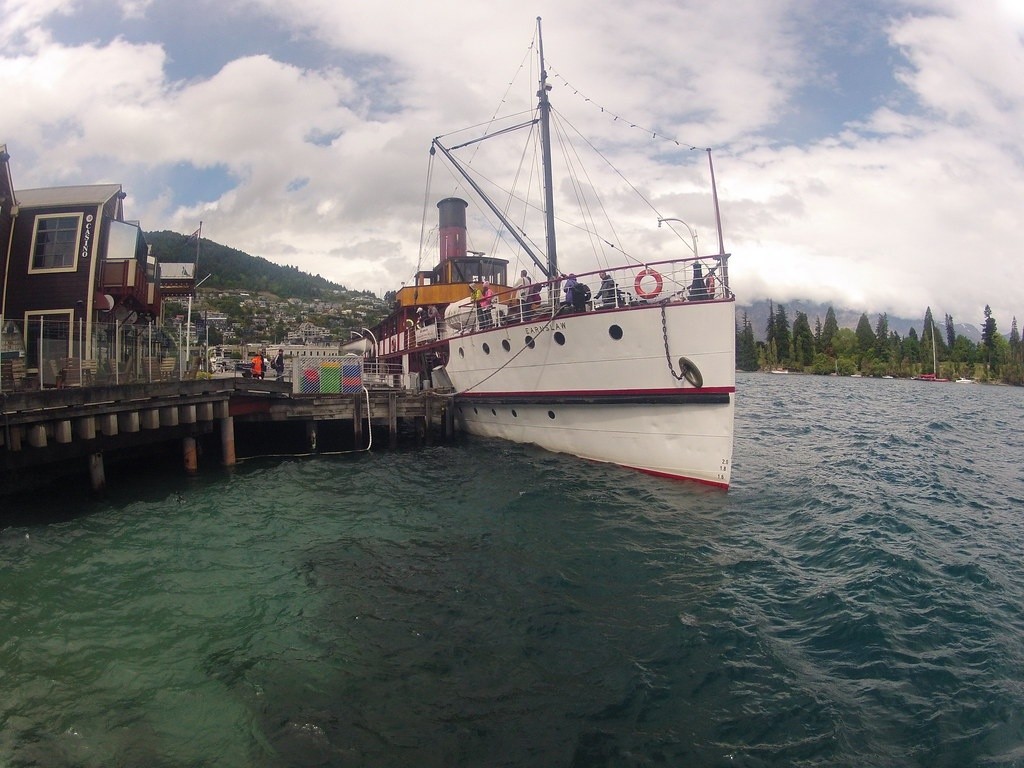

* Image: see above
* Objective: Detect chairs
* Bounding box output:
[534,298,673,320]
[2,356,202,393]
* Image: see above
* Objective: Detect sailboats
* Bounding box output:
[339,16,741,497]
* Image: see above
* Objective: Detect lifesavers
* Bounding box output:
[634,269,664,299]
[706,275,715,299]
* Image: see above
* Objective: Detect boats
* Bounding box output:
[771,366,806,375]
[955,377,974,383]
[851,374,863,378]
[883,375,894,379]
[911,374,948,382]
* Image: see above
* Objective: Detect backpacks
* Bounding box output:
[271,355,278,369]
[573,283,586,312]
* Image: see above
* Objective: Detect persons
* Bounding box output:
[469,283,484,331]
[564,273,579,304]
[594,271,619,309]
[427,303,441,341]
[480,281,494,330]
[511,270,531,322]
[271,349,286,381]
[252,350,265,380]
[416,307,432,327]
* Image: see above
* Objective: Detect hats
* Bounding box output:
[416,307,423,313]
[279,349,284,352]
[483,281,490,287]
